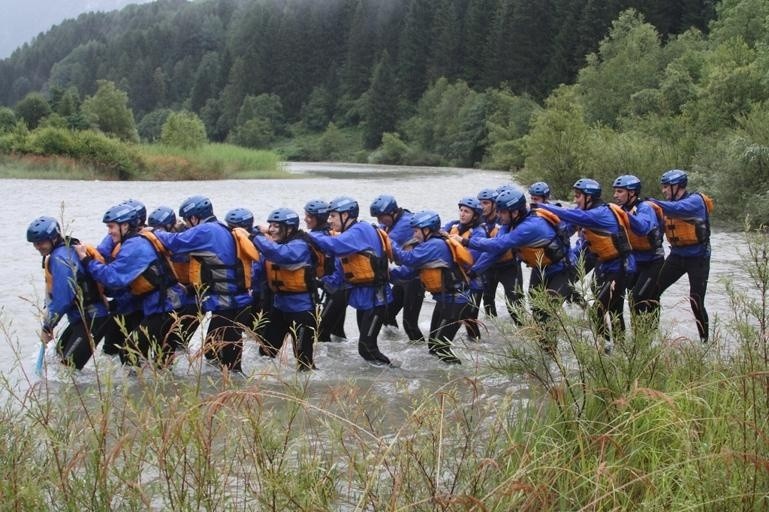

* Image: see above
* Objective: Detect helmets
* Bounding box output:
[225,208,253,227]
[410,211,440,230]
[528,182,550,196]
[661,170,687,188]
[495,190,526,210]
[179,195,213,219]
[267,208,299,227]
[304,199,328,219]
[118,199,146,220]
[370,194,396,217]
[613,175,641,190]
[478,188,499,202]
[573,178,601,199]
[27,216,60,242]
[458,197,483,215]
[103,204,137,225]
[496,185,515,195]
[326,197,359,217]
[148,206,176,227]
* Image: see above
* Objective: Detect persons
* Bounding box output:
[28,176,663,374]
[647,169,711,342]
